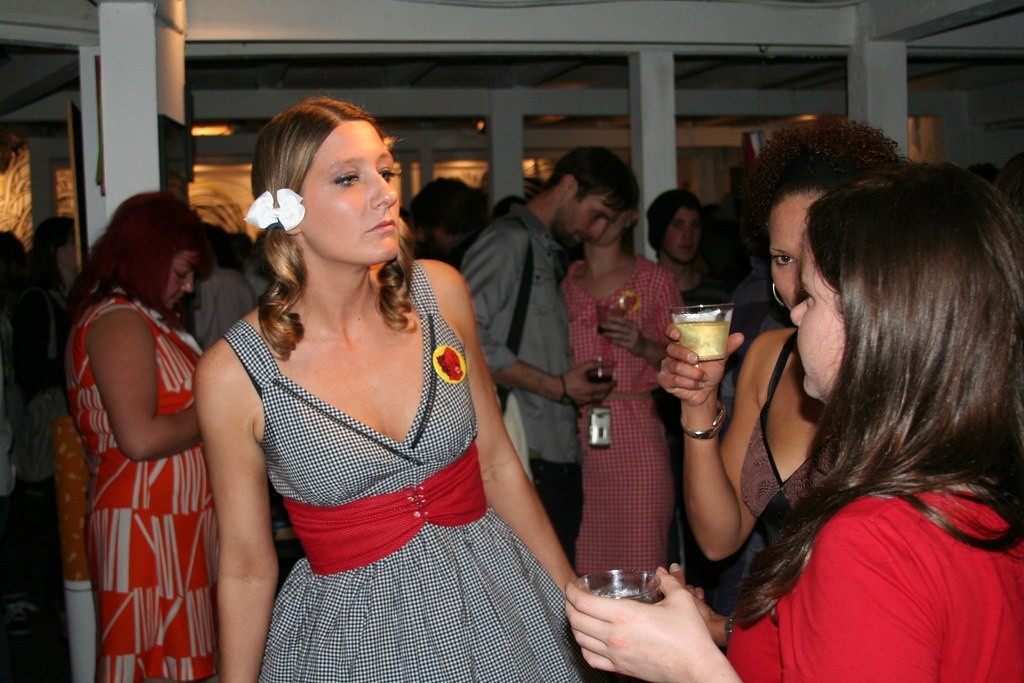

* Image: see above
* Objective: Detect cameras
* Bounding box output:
[589,407,611,446]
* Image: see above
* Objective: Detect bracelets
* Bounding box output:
[725,614,734,644]
[559,374,573,404]
[681,400,726,439]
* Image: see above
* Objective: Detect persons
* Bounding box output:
[0,190,270,683]
[401,144,751,591]
[562,160,1023,683]
[194,95,614,683]
[654,115,909,653]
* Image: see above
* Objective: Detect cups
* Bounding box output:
[672,303,734,361]
[597,302,628,338]
[585,344,614,405]
[574,569,663,604]
